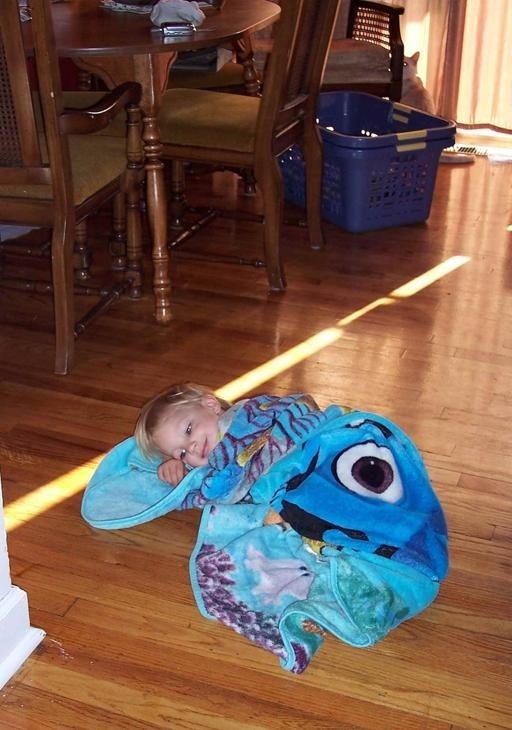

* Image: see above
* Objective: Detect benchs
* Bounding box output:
[256,0,406,101]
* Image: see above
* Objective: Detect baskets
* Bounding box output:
[272,86,458,235]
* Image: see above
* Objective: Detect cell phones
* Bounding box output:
[150,22,195,36]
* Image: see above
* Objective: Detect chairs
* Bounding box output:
[0,0,345,379]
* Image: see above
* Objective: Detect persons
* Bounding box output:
[130,380,233,490]
